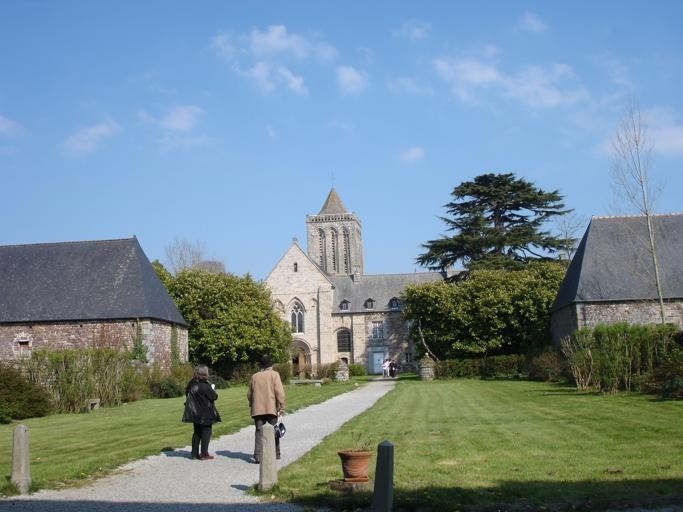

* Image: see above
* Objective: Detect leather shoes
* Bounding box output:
[255,460,260,463]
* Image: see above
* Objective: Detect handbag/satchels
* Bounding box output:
[275,423,286,439]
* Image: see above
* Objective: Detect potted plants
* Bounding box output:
[338,429,377,481]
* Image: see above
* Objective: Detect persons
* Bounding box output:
[247,354,285,463]
[182,364,220,460]
[381,359,396,378]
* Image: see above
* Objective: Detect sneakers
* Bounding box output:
[193,454,215,459]
